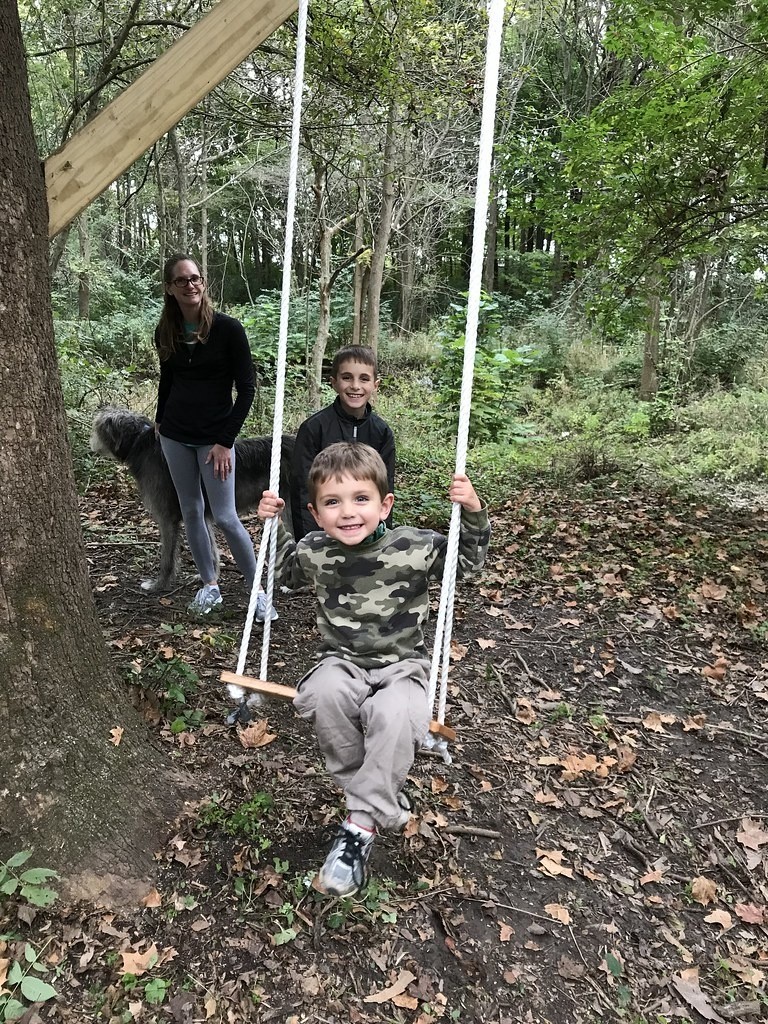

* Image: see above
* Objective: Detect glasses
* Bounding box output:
[170,276,204,287]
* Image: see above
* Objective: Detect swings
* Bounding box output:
[218,0,506,742]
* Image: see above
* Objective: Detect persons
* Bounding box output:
[257,444,491,903]
[155,255,279,623]
[290,346,396,545]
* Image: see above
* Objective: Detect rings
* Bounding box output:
[225,465,228,467]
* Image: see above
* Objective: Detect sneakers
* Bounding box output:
[391,791,411,833]
[187,584,223,616]
[255,594,278,622]
[320,813,377,899]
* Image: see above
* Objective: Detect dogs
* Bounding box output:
[88,409,299,595]
